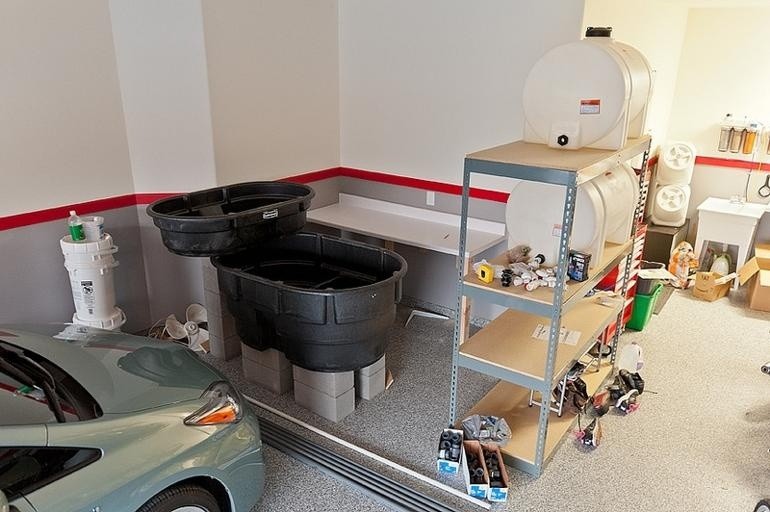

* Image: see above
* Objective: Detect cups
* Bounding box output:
[718,126,758,153]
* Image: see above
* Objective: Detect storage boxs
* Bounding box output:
[738,243,770,313]
[625,280,664,332]
[688,271,732,304]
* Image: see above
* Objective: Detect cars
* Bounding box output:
[0,322,266,512]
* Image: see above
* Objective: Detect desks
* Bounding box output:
[303,193,509,349]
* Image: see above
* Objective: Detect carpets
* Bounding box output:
[654,282,676,318]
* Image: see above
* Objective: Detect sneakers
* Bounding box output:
[616,388,639,413]
[619,368,644,394]
[575,418,602,446]
[606,374,629,399]
[561,376,588,401]
[553,382,586,414]
[591,390,611,416]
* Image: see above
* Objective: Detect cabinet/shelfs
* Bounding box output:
[447,136,653,479]
[644,218,690,272]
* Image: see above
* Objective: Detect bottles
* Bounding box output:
[68,210,86,242]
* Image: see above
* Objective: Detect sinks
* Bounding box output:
[692,189,767,292]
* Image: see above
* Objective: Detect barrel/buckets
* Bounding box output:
[57,233,119,321]
[70,306,128,334]
